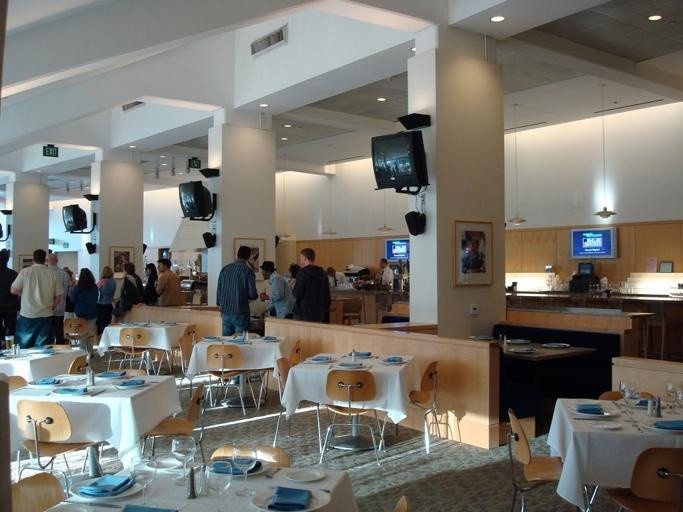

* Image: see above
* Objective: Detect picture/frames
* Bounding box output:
[232,237,265,283]
[17,253,33,274]
[448,216,494,288]
[107,244,135,280]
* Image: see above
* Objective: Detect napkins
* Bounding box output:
[268,485,312,511]
[386,357,402,363]
[78,474,134,497]
[348,351,372,356]
[339,363,363,368]
[576,404,604,416]
[208,459,261,474]
[635,399,659,407]
[312,356,330,361]
[655,420,683,431]
[122,504,179,512]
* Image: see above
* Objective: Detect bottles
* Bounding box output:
[547,273,569,292]
[511,281,517,291]
[388,280,392,291]
[186,466,209,499]
[668,286,682,297]
[587,283,638,296]
[351,348,356,363]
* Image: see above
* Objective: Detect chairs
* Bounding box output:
[1,317,302,418]
[16,399,104,495]
[11,472,67,512]
[508,407,591,512]
[392,494,411,512]
[210,443,289,468]
[378,360,441,455]
[141,383,208,466]
[273,357,334,454]
[320,369,386,465]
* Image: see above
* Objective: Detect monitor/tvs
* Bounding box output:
[62,204,88,232]
[385,239,410,262]
[0,224,3,239]
[371,130,430,191]
[179,180,212,219]
[570,227,617,260]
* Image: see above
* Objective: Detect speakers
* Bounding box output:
[143,244,147,254]
[275,235,279,247]
[86,243,95,254]
[405,211,425,235]
[203,232,215,248]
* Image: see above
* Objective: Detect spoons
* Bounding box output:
[264,467,282,479]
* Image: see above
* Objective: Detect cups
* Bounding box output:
[4,336,13,348]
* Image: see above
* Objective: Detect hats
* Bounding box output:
[259,261,277,273]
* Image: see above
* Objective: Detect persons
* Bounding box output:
[326,266,348,288]
[376,258,394,291]
[259,260,296,322]
[292,248,331,324]
[214,245,258,383]
[0,244,184,350]
[288,263,301,284]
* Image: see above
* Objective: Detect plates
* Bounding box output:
[251,488,331,512]
[0,344,46,360]
[66,478,142,500]
[283,467,326,483]
[468,339,571,355]
[146,456,182,471]
[117,320,175,329]
[206,456,271,478]
[301,353,406,372]
[199,335,280,345]
[567,396,682,434]
[28,370,148,396]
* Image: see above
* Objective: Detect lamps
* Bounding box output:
[505,103,527,227]
[275,170,290,242]
[590,83,617,220]
[375,188,392,232]
[319,161,337,236]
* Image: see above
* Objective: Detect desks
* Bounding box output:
[169,291,410,330]
[554,388,683,512]
[43,457,348,512]
[290,353,415,452]
[9,374,174,490]
[466,290,682,437]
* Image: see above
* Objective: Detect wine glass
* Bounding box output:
[665,380,676,414]
[618,379,639,414]
[622,390,640,422]
[129,455,160,508]
[229,443,259,500]
[672,384,682,413]
[204,461,234,512]
[170,435,196,485]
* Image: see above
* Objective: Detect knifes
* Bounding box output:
[58,499,123,510]
